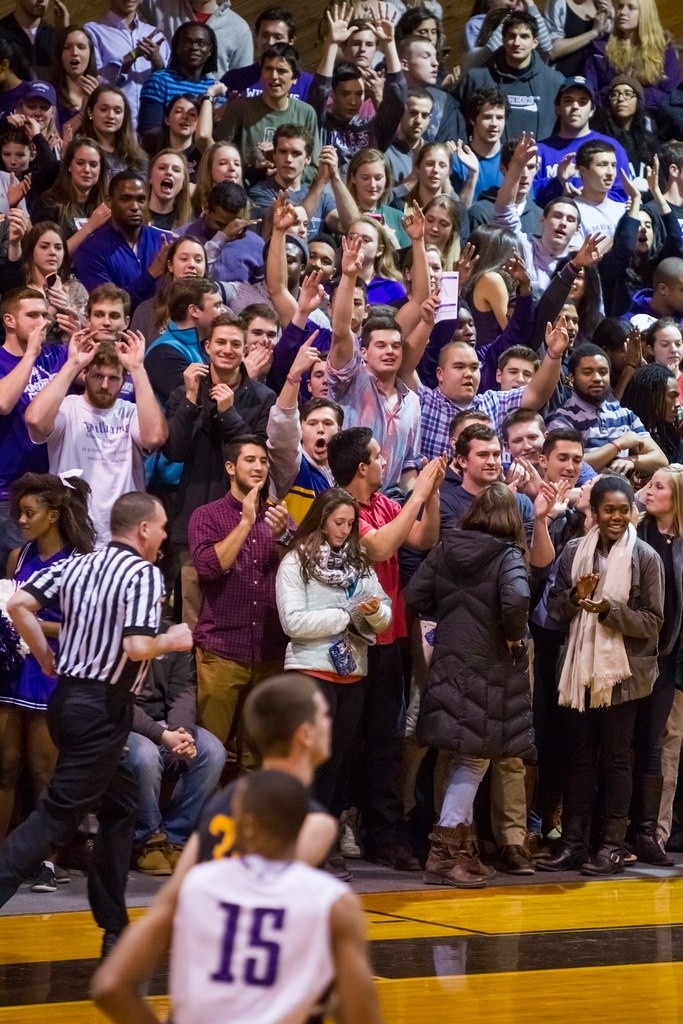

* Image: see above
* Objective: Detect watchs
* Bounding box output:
[201,96,213,102]
[273,530,293,546]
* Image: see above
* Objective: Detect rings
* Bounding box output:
[591,607,596,611]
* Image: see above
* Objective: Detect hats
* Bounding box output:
[262,233,310,271]
[557,75,596,102]
[606,73,644,99]
[24,80,56,104]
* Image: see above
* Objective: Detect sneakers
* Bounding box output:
[100,932,117,958]
[30,859,73,893]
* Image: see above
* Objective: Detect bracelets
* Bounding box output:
[284,375,301,383]
[79,112,85,119]
[129,50,136,61]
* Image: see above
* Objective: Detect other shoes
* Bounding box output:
[633,830,683,866]
[475,834,535,874]
[371,833,422,872]
[136,832,183,875]
[524,826,563,859]
[622,850,637,867]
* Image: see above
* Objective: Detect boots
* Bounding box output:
[423,820,498,888]
[321,807,364,881]
[535,815,632,876]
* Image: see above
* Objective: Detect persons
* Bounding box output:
[0,0,683,888]
[92,770,381,1023]
[175,674,337,868]
[0,492,192,966]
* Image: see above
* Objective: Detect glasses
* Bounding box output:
[181,38,210,49]
[610,89,637,99]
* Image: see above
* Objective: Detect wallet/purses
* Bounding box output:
[330,633,358,680]
[514,644,524,659]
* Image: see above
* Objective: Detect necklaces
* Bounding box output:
[129,235,139,251]
